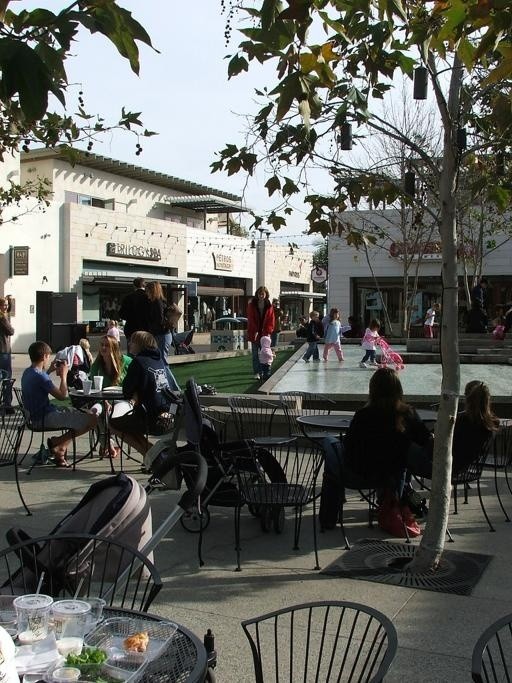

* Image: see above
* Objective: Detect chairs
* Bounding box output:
[200,404,231,451]
[0,378,16,408]
[229,443,330,570]
[0,534,163,614]
[280,390,337,478]
[13,385,76,474]
[333,440,411,550]
[463,418,512,522]
[115,387,180,472]
[0,405,32,517]
[410,425,497,543]
[472,613,512,683]
[241,601,398,683]
[228,395,299,481]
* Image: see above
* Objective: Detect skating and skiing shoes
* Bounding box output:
[324,356,328,360]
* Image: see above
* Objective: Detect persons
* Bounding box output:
[376,379,500,505]
[424,303,439,338]
[462,301,488,334]
[318,367,429,530]
[358,319,381,368]
[144,280,174,360]
[106,331,183,470]
[245,286,275,379]
[89,334,134,458]
[301,311,321,364]
[258,336,276,381]
[269,298,283,347]
[117,277,149,355]
[296,316,310,339]
[470,279,488,310]
[321,308,351,363]
[0,297,16,416]
[21,340,100,467]
[106,320,121,347]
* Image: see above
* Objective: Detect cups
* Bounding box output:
[15,595,47,639]
[51,599,91,655]
[95,375,104,390]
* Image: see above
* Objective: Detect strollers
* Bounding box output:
[374,337,406,370]
[0,449,208,608]
[144,377,285,536]
[171,326,196,355]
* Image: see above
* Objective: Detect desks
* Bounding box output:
[296,415,384,548]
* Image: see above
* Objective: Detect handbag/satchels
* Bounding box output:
[405,485,426,515]
[145,439,183,491]
[32,447,49,465]
[381,492,419,536]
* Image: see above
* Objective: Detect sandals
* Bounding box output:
[99,447,109,457]
[56,449,67,466]
[110,449,117,458]
[47,436,56,454]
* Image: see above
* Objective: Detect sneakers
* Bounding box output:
[359,361,366,368]
[370,362,380,366]
[260,372,264,378]
[338,358,344,361]
[254,373,260,379]
[313,359,320,362]
[302,356,310,362]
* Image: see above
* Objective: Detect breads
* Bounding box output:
[125,630,151,654]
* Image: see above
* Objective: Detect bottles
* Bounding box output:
[52,599,92,664]
[12,594,54,647]
[94,376,104,392]
